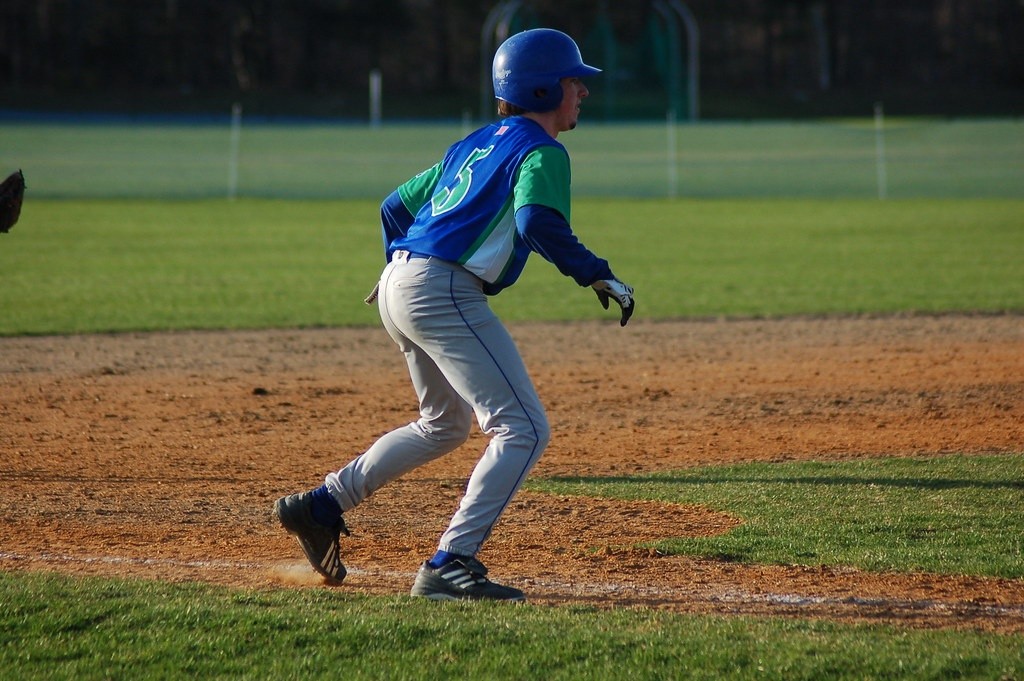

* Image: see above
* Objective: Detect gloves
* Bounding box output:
[591,277,634,326]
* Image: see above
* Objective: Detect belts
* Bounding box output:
[398,252,431,259]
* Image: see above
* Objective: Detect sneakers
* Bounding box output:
[410,557,524,603]
[273,489,351,586]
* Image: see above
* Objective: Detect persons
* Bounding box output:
[274,28,635,604]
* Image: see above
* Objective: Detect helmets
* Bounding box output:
[491,28,602,112]
